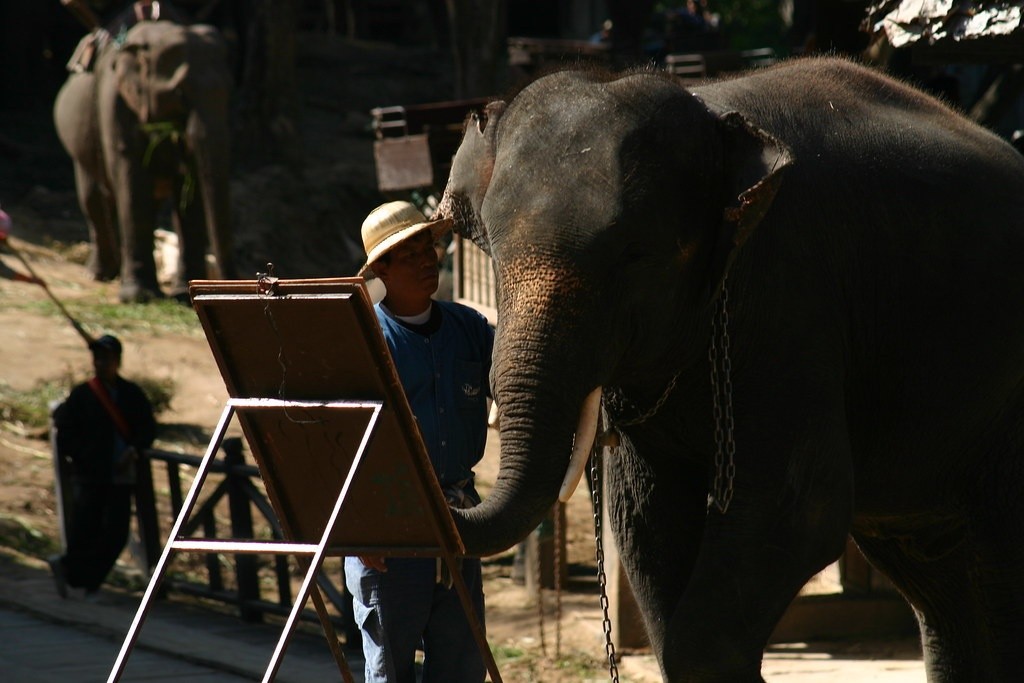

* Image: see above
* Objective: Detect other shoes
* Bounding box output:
[84,582,98,597]
[48,555,68,594]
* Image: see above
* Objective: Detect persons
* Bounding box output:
[45,335,157,598]
[342,200,492,683]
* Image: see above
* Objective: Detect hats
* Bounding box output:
[357,200,454,282]
[88,336,121,356]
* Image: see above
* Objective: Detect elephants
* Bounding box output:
[435,53,1023,683]
[53,21,235,307]
